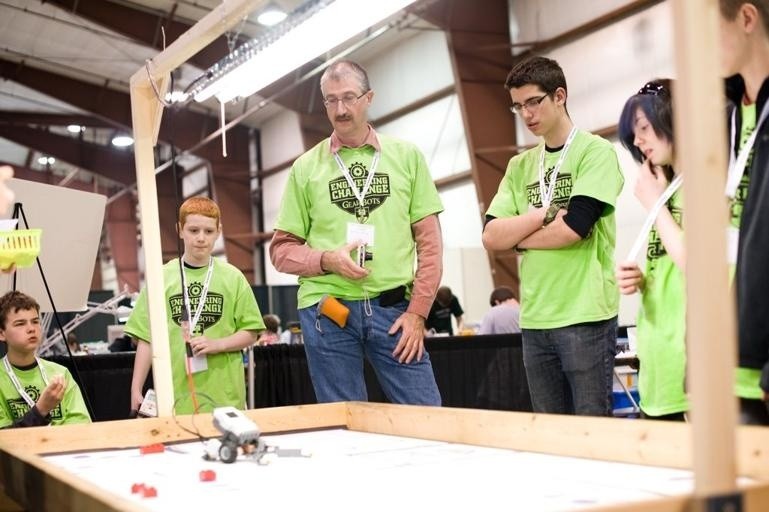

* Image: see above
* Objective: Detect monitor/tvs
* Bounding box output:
[250,286,269,317]
[272,285,300,334]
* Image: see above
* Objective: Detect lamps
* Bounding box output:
[185,0,417,107]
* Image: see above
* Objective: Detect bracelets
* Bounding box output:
[513,245,528,254]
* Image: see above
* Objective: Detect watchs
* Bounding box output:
[542,205,560,226]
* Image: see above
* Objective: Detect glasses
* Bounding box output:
[508,89,555,113]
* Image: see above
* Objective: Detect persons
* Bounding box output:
[617,79,689,424]
[423,286,464,338]
[478,285,522,335]
[480,55,623,417]
[674,1,768,425]
[268,61,446,406]
[63,314,305,365]
[124,197,267,414]
[1,290,92,431]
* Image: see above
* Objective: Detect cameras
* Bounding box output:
[357,243,373,267]
